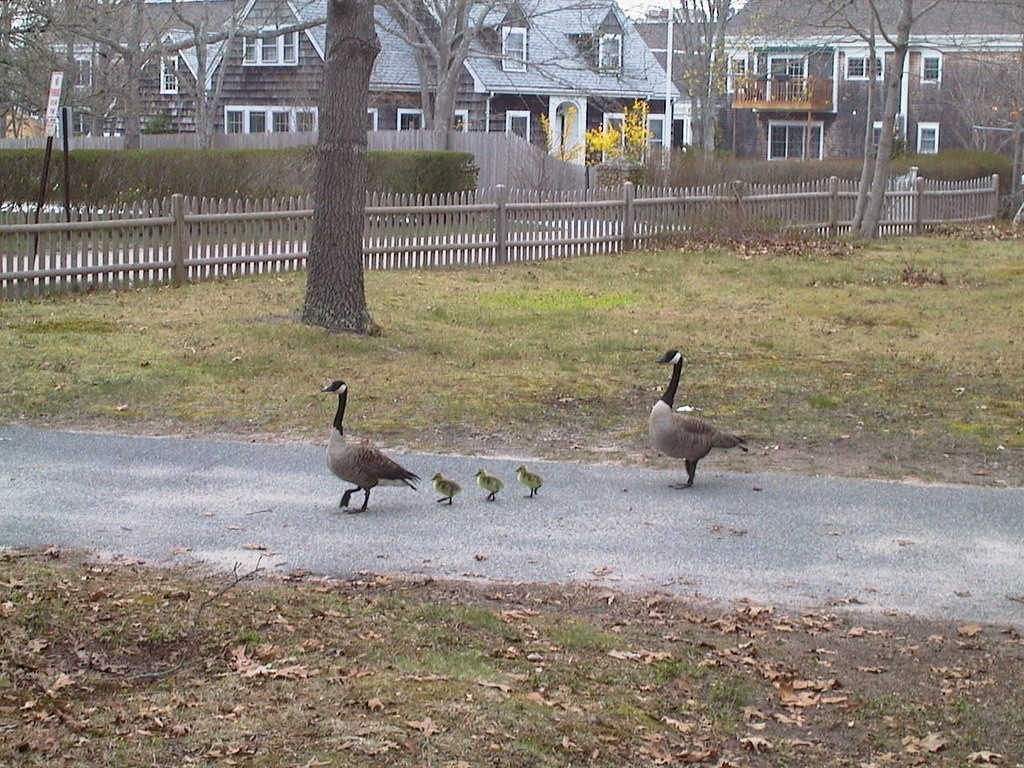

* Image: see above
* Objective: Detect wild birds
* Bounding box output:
[514,465,544,498]
[321,385,420,515]
[474,469,504,502]
[649,349,747,491]
[431,474,462,506]
